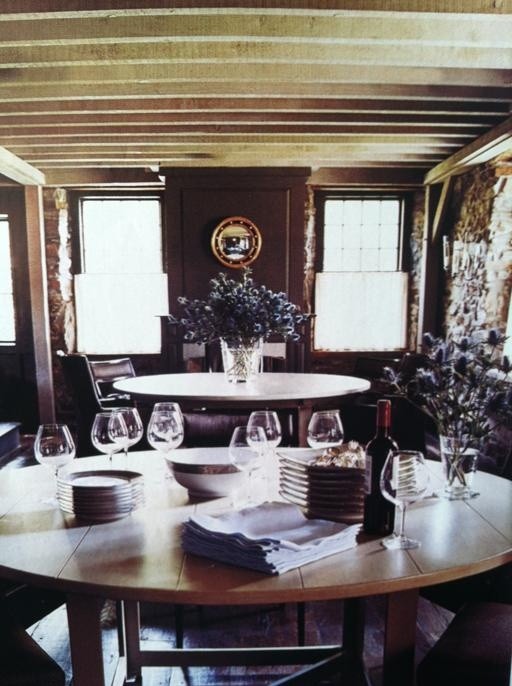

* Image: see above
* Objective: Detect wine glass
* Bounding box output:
[34,400,185,504]
[380,449,429,551]
[245,410,283,494]
[228,425,267,506]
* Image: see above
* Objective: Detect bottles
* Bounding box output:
[364,399,400,535]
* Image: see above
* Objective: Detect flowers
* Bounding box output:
[382,330,511,438]
[167,272,315,349]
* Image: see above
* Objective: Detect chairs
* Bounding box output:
[60,350,140,452]
[169,407,306,647]
[417,597,511,685]
[340,354,406,450]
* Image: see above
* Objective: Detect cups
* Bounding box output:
[305,408,346,450]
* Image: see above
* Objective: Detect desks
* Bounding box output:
[0,452,510,685]
[110,369,374,447]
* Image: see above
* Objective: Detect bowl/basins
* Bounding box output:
[167,451,244,499]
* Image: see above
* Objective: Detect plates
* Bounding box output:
[56,463,145,520]
[273,446,365,522]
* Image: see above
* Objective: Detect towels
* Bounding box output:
[178,497,362,578]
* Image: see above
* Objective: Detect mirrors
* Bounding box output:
[209,213,263,267]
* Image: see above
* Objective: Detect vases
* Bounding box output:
[434,428,485,501]
[216,332,264,385]
